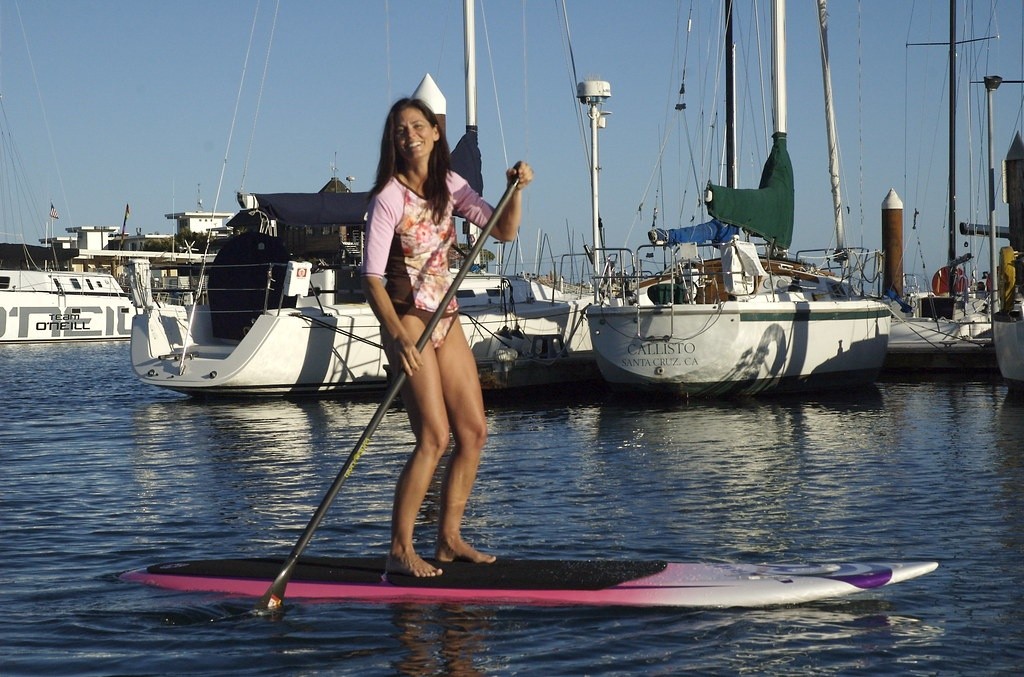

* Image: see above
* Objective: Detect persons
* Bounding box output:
[363,96,534,576]
[517,272,551,283]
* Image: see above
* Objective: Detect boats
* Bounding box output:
[0,198,187,342]
[116,555,939,607]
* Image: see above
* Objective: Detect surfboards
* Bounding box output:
[113,559,940,605]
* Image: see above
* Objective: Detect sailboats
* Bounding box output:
[129,0,596,394]
[586,0,891,402]
[886,0,1024,379]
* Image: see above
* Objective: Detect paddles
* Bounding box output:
[257,178,520,612]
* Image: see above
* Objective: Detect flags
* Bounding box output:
[50,202,59,219]
